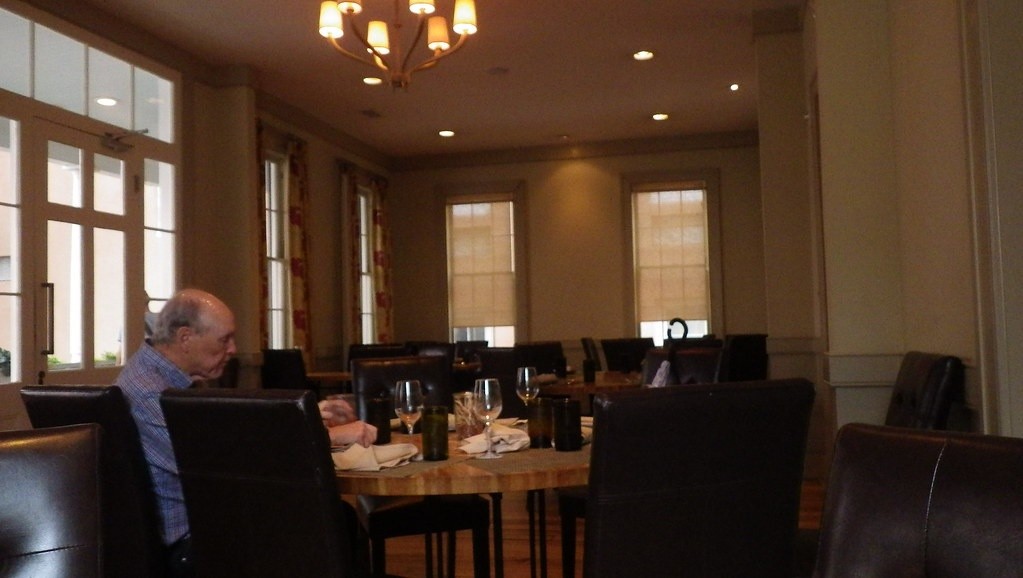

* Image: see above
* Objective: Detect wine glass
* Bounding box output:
[395,381,424,461]
[510,367,539,427]
[474,379,503,459]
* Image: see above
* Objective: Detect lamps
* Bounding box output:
[319,0,477,96]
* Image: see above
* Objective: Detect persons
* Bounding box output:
[109,287,378,578]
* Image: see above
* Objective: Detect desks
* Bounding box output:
[328,418,590,578]
[306,371,353,399]
[540,371,674,399]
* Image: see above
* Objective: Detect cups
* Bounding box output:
[355,372,375,420]
[526,398,552,448]
[553,399,579,452]
[365,399,391,444]
[583,359,595,382]
[453,392,483,438]
[424,405,448,460]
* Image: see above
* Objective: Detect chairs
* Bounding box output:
[0,333,1023,578]
